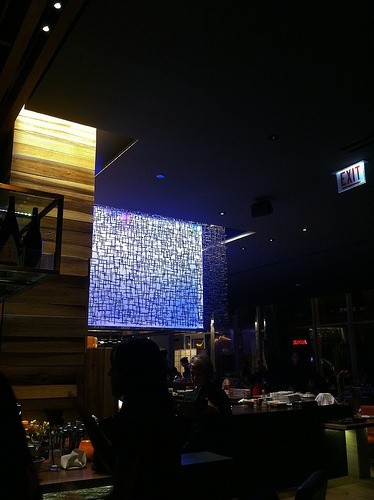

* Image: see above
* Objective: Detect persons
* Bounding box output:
[180,357,189,374]
[90,337,185,500]
[214,331,231,354]
[177,354,230,422]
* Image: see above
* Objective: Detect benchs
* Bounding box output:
[358,406,374,444]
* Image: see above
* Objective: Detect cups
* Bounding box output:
[168,388,173,393]
[79,440,94,460]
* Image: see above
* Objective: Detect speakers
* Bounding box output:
[251,201,274,218]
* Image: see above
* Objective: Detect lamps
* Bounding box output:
[248,198,273,218]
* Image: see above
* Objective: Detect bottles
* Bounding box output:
[51,416,86,456]
[254,400,258,408]
[261,390,266,404]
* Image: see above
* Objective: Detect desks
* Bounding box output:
[35,449,114,493]
[320,420,374,479]
[215,404,309,423]
[174,398,193,419]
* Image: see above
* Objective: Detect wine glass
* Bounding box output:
[31,432,42,458]
